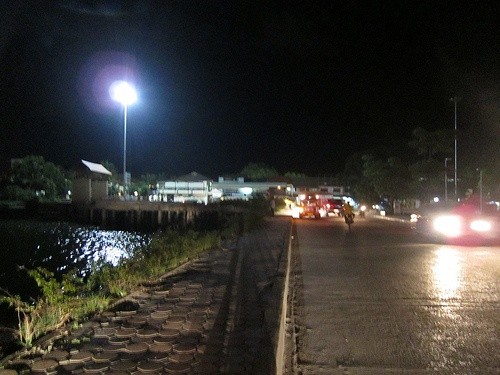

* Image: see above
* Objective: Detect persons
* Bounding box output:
[343,202,355,222]
[269,197,275,217]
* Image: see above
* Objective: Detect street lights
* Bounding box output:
[442,156,453,208]
[110,81,137,199]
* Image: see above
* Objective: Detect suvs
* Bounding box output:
[300,196,321,220]
[327,198,343,218]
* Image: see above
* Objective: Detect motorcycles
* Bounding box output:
[344,212,355,228]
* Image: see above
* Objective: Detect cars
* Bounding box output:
[415,209,500,245]
